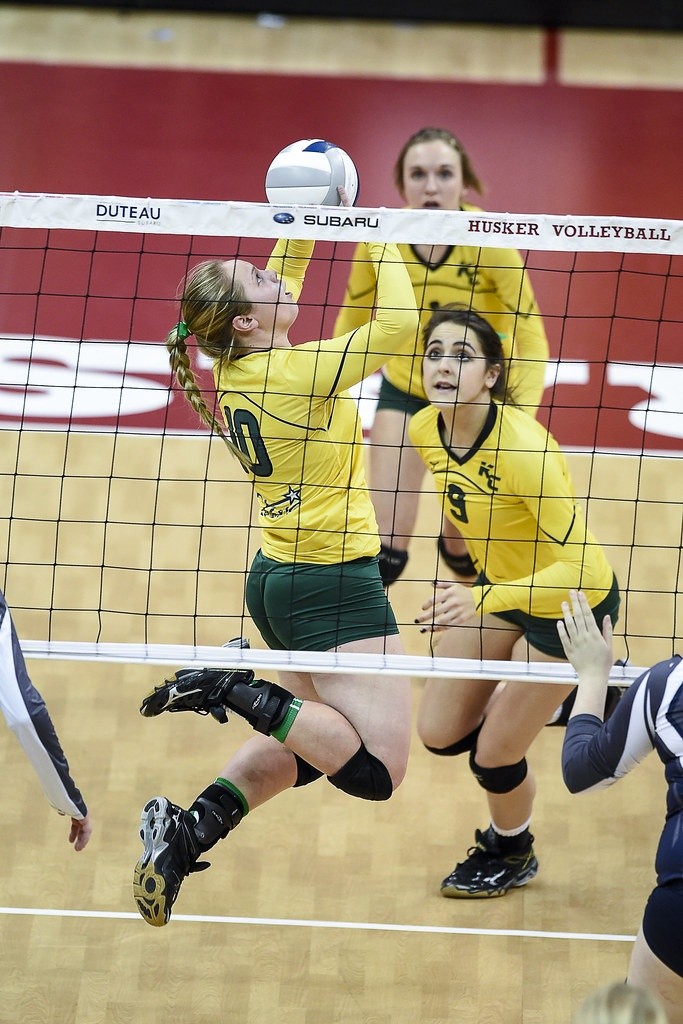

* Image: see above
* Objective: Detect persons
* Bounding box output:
[134,186,411,926]
[331,126,550,593]
[0,587,92,851]
[557,590,683,1024]
[409,302,630,902]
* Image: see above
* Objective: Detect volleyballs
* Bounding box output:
[265,138,359,208]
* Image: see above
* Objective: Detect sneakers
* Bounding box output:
[132,796,211,928]
[441,823,538,897]
[138,635,255,723]
